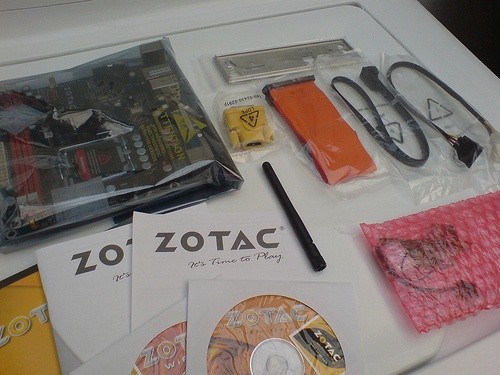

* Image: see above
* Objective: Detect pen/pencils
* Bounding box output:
[261,161,328,272]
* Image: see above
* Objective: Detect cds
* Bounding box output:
[204,293,349,375]
[131,322,187,375]
